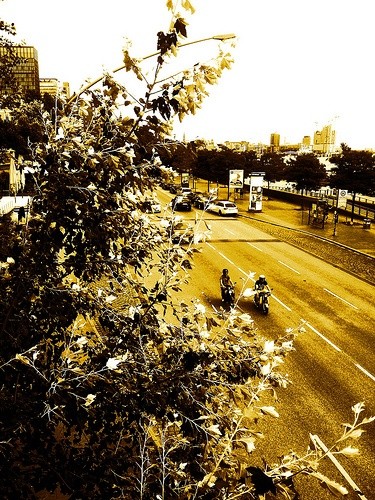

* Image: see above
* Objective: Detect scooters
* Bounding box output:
[252,288,274,315]
[220,282,237,309]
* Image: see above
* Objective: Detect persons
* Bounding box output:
[220,269,235,302]
[254,275,271,305]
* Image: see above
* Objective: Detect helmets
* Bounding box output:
[223,269,228,274]
[259,274,265,281]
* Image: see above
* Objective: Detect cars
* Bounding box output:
[165,219,195,244]
[206,200,239,217]
[156,178,179,194]
[172,195,192,211]
[179,187,210,210]
